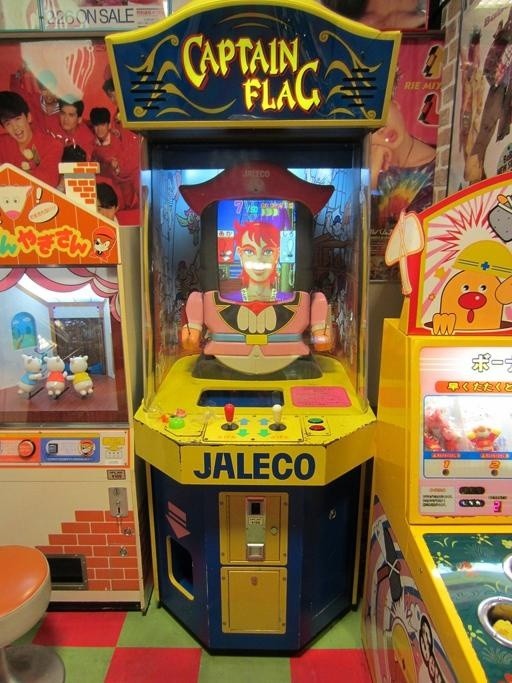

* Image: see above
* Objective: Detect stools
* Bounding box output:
[1,544,65,683]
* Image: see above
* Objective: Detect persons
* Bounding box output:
[0,57,139,226]
[369,95,435,280]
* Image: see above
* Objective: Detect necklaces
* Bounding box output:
[399,135,414,168]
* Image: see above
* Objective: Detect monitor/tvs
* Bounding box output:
[200,198,313,303]
